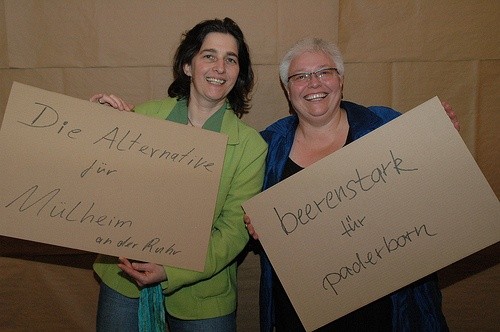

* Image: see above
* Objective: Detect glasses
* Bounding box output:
[287,67,339,85]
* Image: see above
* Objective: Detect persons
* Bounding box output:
[240,36,460,332]
[83,14,268,332]
